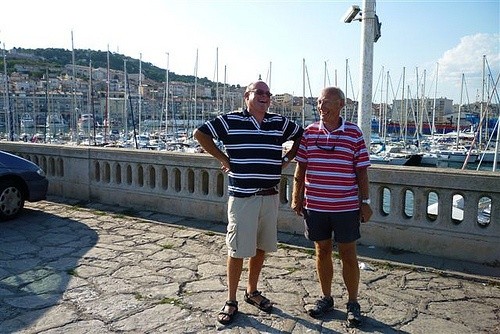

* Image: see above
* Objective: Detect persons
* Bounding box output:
[193,81,305,325]
[290,86,373,328]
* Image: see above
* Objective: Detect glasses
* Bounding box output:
[248,89,272,97]
[315,132,340,151]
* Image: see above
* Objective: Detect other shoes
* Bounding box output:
[309,296,334,317]
[346,301,361,327]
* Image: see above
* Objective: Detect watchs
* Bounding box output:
[283,156,291,164]
[362,199,370,205]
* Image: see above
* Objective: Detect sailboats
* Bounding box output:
[0,27,500,226]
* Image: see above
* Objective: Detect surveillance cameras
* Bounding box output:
[343,4,360,23]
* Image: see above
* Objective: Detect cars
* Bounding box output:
[0,151,49,225]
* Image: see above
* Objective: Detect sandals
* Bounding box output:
[243,289,273,311]
[217,300,238,325]
